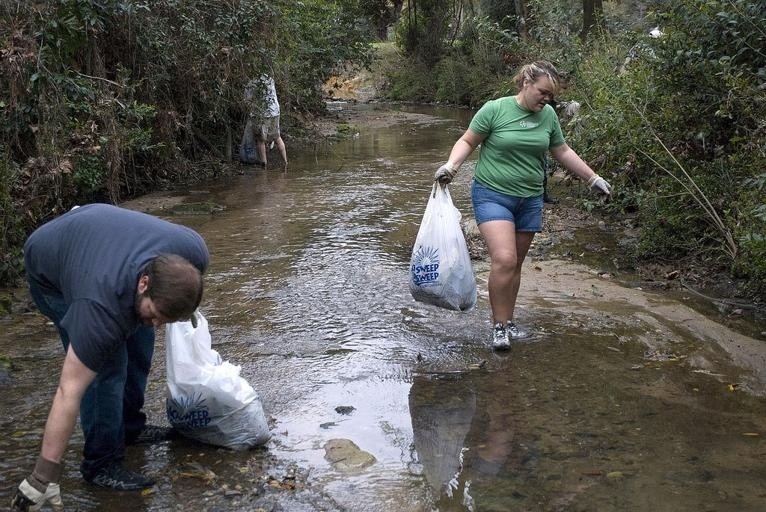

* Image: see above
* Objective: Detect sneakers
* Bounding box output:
[128,422,173,446]
[88,461,156,492]
[490,318,529,352]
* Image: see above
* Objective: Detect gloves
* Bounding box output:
[586,173,613,206]
[8,454,66,512]
[434,162,458,185]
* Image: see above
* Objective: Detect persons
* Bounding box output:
[243,70,289,167]
[432,57,614,351]
[541,71,583,205]
[617,25,668,75]
[11,199,211,512]
